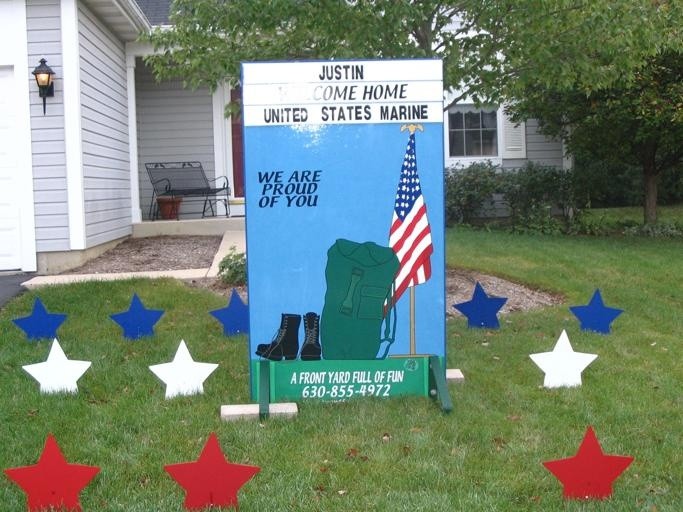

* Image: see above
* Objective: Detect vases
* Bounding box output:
[155,194,182,219]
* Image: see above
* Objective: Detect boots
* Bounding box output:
[301,312,321,359]
[255,314,301,360]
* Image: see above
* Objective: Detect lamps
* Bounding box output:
[30,57,56,115]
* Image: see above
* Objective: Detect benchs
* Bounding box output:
[143,159,232,220]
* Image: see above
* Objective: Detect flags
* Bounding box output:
[383,134,434,318]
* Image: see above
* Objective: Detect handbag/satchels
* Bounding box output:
[320,239,400,359]
